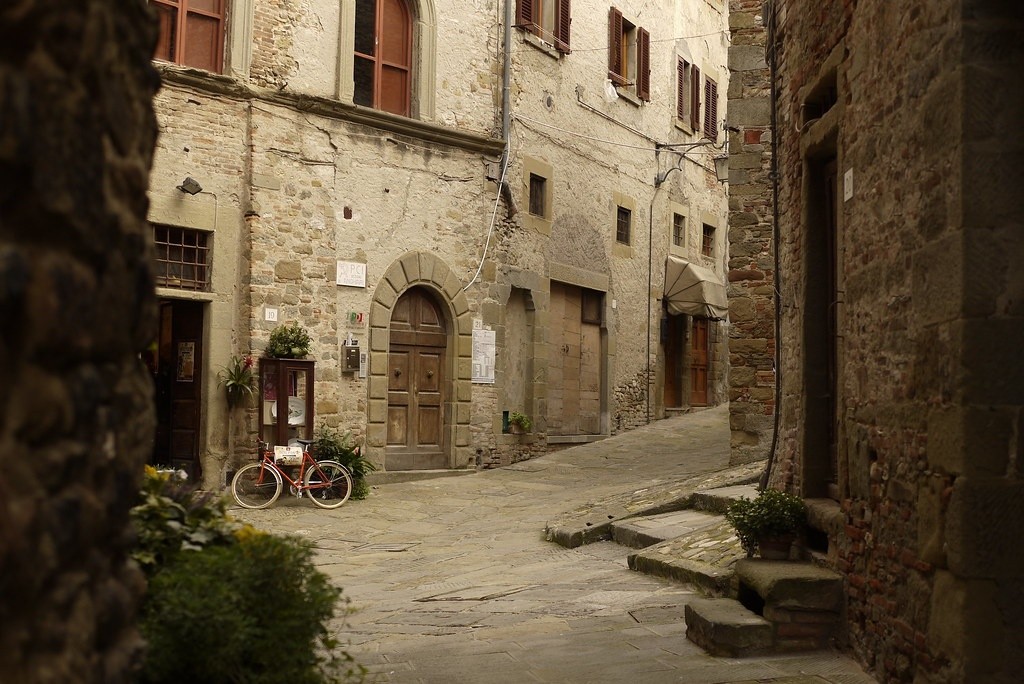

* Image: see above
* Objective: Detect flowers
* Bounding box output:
[215,354,261,407]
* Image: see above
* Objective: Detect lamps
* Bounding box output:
[176,177,203,196]
[711,150,728,186]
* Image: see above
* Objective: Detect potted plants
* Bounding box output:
[340,476,371,500]
[726,489,809,561]
[312,420,376,494]
[263,319,316,359]
[508,410,534,435]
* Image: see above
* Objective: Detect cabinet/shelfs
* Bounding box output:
[257,357,317,495]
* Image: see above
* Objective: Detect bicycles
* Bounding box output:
[231,431,355,510]
